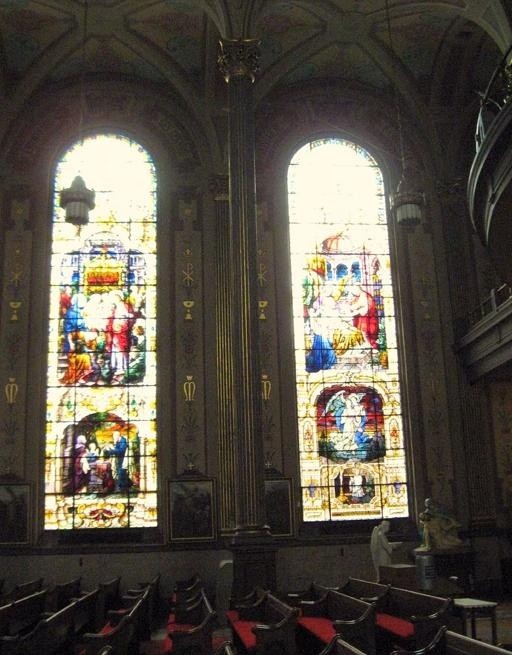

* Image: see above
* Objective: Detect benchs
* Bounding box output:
[1,574,512,655]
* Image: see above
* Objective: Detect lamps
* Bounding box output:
[55,2,96,226]
[379,2,424,226]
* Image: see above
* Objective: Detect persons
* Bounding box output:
[370,520,392,582]
[418,512,463,545]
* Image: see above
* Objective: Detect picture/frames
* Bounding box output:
[1,479,36,551]
[264,476,297,544]
[162,471,217,549]
